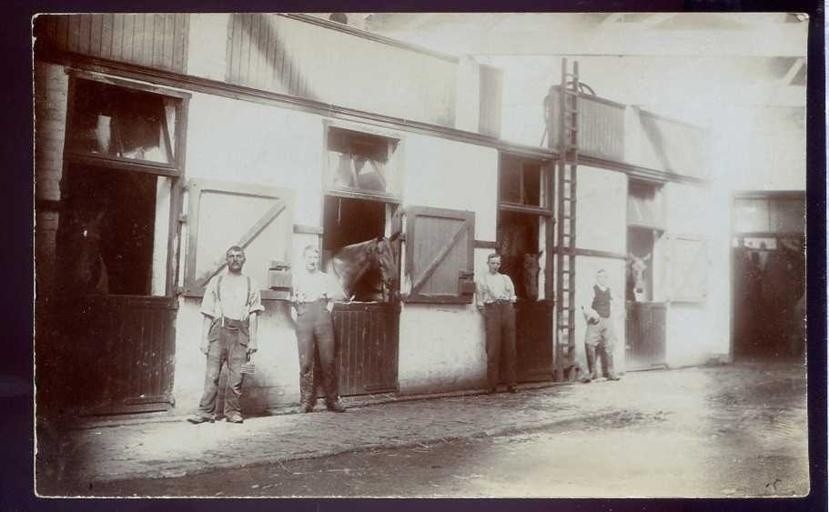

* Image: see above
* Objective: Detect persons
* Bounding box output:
[475,254,519,393]
[582,269,620,383]
[286,244,345,413]
[187,246,266,424]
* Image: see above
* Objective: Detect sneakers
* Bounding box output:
[187,412,215,423]
[583,370,621,382]
[226,413,243,423]
[299,401,347,412]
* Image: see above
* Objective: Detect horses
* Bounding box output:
[628,252,652,303]
[324,230,406,302]
[500,247,545,303]
[71,211,110,295]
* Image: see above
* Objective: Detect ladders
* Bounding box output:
[554,57,580,380]
